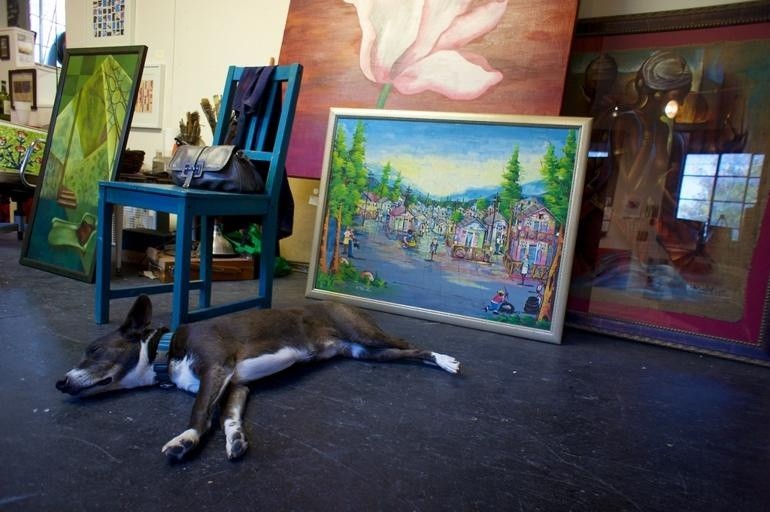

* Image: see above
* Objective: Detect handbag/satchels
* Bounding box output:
[167,137,265,194]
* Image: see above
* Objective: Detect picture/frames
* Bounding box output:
[305,108,593,346]
[560,1,770,367]
[9,69,36,109]
[19,45,149,284]
[0,119,49,177]
[129,64,166,129]
[0,36,9,59]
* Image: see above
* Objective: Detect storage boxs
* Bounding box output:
[145,246,254,283]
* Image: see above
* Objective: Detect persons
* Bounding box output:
[575,50,720,284]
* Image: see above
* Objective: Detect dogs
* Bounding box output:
[54,293,463,466]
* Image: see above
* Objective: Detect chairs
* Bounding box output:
[94,62,303,331]
